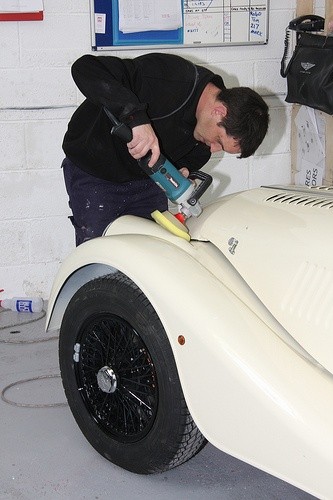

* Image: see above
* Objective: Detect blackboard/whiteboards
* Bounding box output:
[89,0,270,52]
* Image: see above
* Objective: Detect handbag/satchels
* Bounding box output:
[284,32,332,116]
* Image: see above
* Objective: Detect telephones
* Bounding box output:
[288,14,325,32]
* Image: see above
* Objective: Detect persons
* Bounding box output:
[62,54,270,247]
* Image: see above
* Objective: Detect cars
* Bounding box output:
[43,185,333,500]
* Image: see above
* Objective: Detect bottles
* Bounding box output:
[0,298,44,313]
